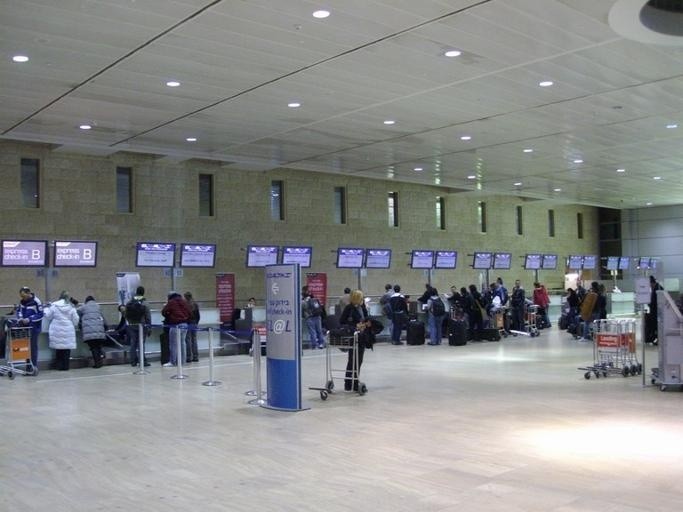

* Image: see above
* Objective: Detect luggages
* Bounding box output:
[448,307,467,346]
[407,318,425,345]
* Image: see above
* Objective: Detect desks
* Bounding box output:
[1,292,637,364]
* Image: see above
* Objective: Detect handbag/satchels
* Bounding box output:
[6,318,24,329]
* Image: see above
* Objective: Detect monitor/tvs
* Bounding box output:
[247,245,279,268]
[135,241,176,268]
[179,243,216,268]
[411,249,659,269]
[282,246,312,268]
[336,248,364,268]
[1,240,48,267]
[365,248,392,269]
[53,239,98,267]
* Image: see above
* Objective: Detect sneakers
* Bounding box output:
[427,342,435,346]
[163,361,174,367]
[391,341,403,346]
[131,360,151,367]
[186,357,199,362]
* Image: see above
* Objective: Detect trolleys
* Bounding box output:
[0,326,38,380]
[484,304,540,340]
[577,318,642,380]
[309,328,367,401]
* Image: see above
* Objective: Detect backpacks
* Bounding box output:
[126,299,143,322]
[306,298,324,316]
[428,297,445,317]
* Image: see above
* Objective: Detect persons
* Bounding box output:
[339,289,373,391]
[161,292,201,367]
[301,285,325,350]
[650,276,664,290]
[44,291,80,369]
[76,295,106,368]
[380,284,408,344]
[565,280,607,342]
[117,286,152,366]
[419,277,551,345]
[13,286,44,371]
[340,289,351,311]
[246,297,256,308]
